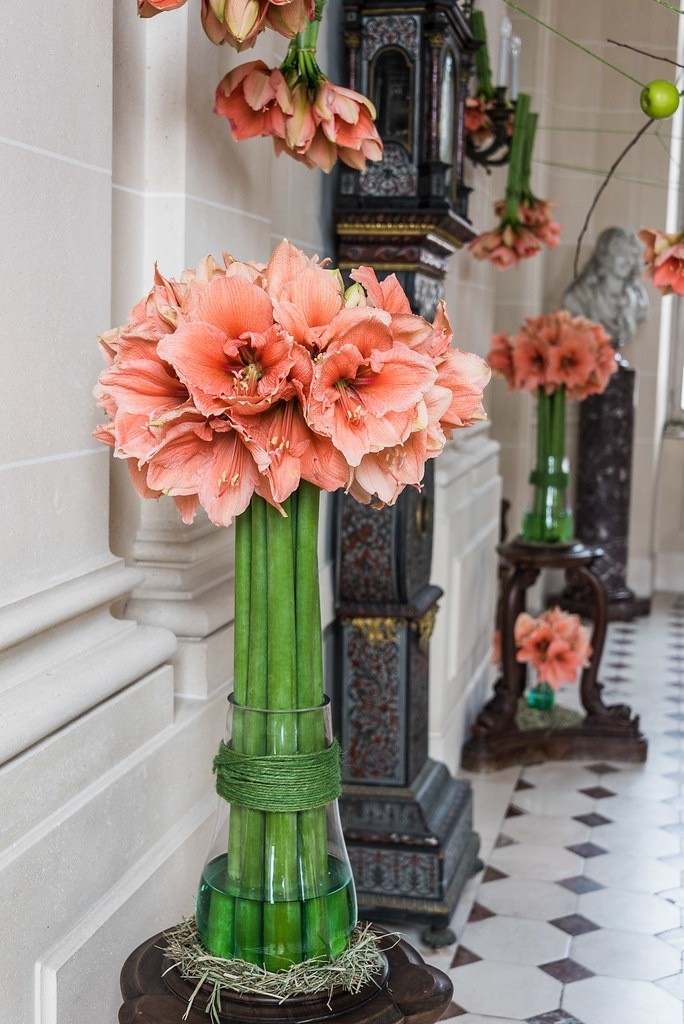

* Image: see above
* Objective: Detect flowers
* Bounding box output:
[90,233,492,973]
[470,91,560,265]
[639,228,684,296]
[486,308,618,538]
[511,607,595,692]
[465,10,518,144]
[137,0,386,176]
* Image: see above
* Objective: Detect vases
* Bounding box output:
[196,692,359,974]
[519,457,577,546]
[526,684,558,714]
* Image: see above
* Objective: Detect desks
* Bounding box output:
[462,536,650,773]
[119,925,453,1024]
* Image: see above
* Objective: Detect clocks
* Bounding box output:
[334,2,488,942]
